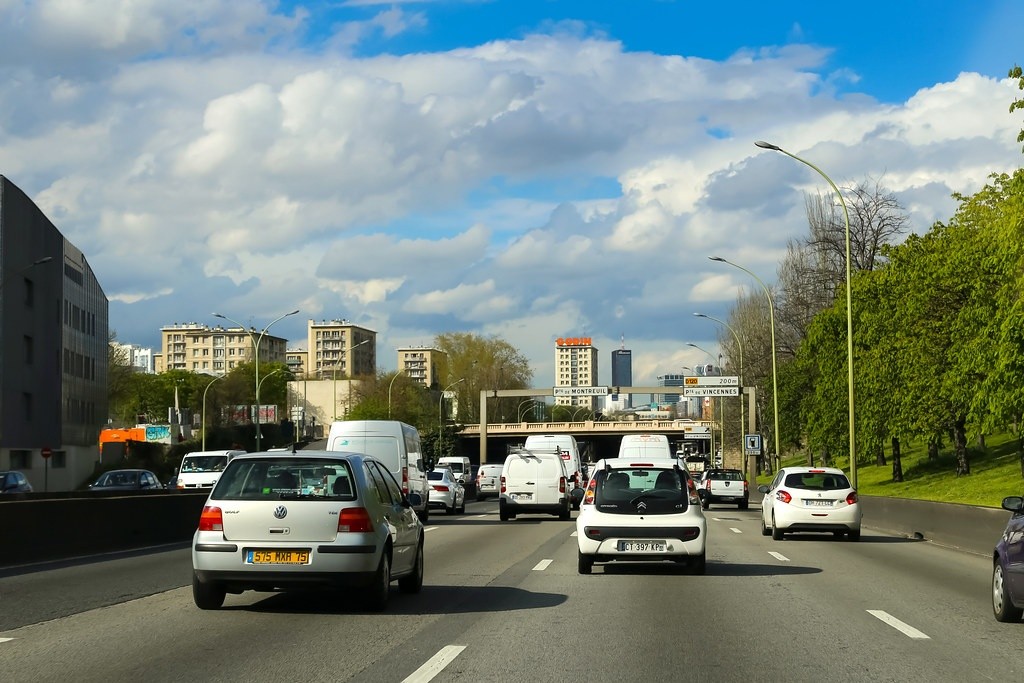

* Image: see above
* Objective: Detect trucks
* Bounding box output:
[686,456,707,478]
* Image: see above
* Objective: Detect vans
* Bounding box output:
[675,450,685,457]
[175,450,246,489]
[618,435,673,459]
[326,419,435,523]
[437,456,505,501]
[498,435,586,520]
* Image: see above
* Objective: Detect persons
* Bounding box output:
[178,433,185,442]
[190,459,197,470]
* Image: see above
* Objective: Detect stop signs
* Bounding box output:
[41,447,53,458]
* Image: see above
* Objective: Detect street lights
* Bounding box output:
[333,339,371,422]
[706,255,782,473]
[685,342,724,469]
[517,398,538,423]
[202,374,228,452]
[681,365,716,466]
[388,364,419,420]
[211,309,299,452]
[692,312,746,479]
[439,378,466,456]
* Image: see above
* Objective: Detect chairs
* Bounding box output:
[656,471,680,488]
[712,473,719,479]
[332,474,349,497]
[270,472,298,496]
[823,477,835,488]
[789,476,805,486]
[604,473,630,497]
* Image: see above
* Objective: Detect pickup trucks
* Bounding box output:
[695,469,750,510]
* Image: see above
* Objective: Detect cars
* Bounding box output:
[757,466,863,542]
[705,447,723,468]
[0,471,34,494]
[992,494,1023,623]
[89,469,178,492]
[575,459,708,575]
[192,449,425,610]
[426,467,466,514]
